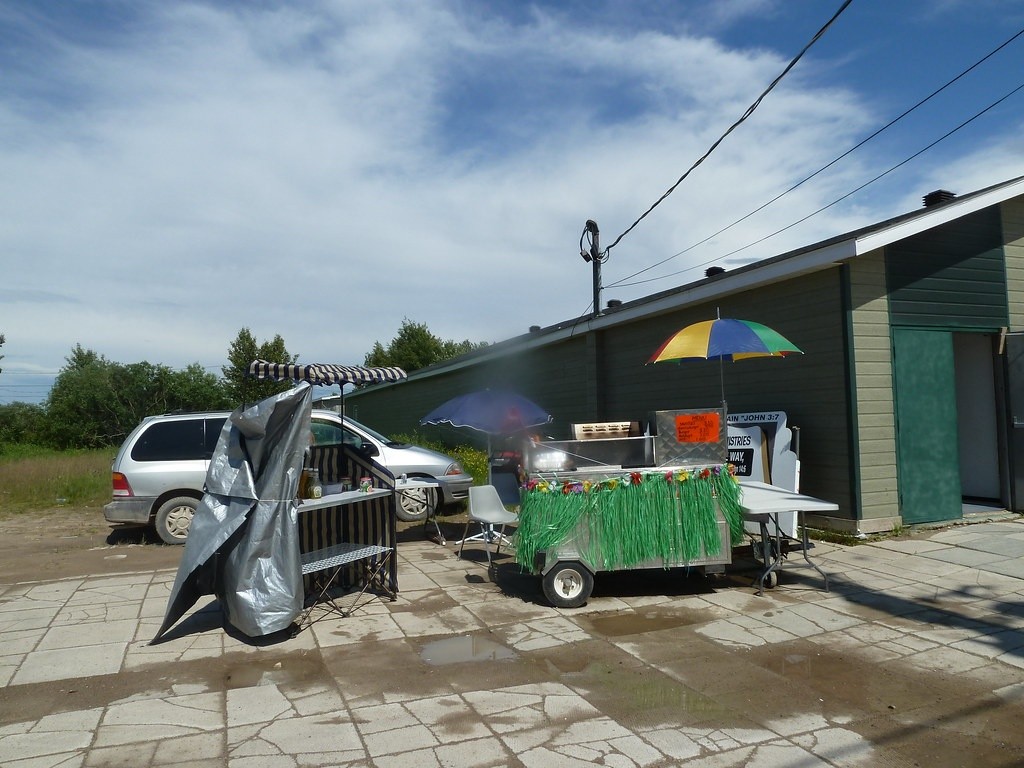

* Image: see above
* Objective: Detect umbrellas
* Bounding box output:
[645,307,804,401]
[419,388,553,544]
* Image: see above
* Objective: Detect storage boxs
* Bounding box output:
[322,482,343,496]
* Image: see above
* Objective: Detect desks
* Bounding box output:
[737,481,839,597]
[395,478,448,546]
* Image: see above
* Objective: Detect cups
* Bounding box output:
[401,471,408,486]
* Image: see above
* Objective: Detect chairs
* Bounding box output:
[457,485,519,569]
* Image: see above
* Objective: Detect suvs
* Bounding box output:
[103,408,475,546]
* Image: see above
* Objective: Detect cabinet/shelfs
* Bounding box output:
[286,488,399,638]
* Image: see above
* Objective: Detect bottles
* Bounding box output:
[300,467,322,499]
